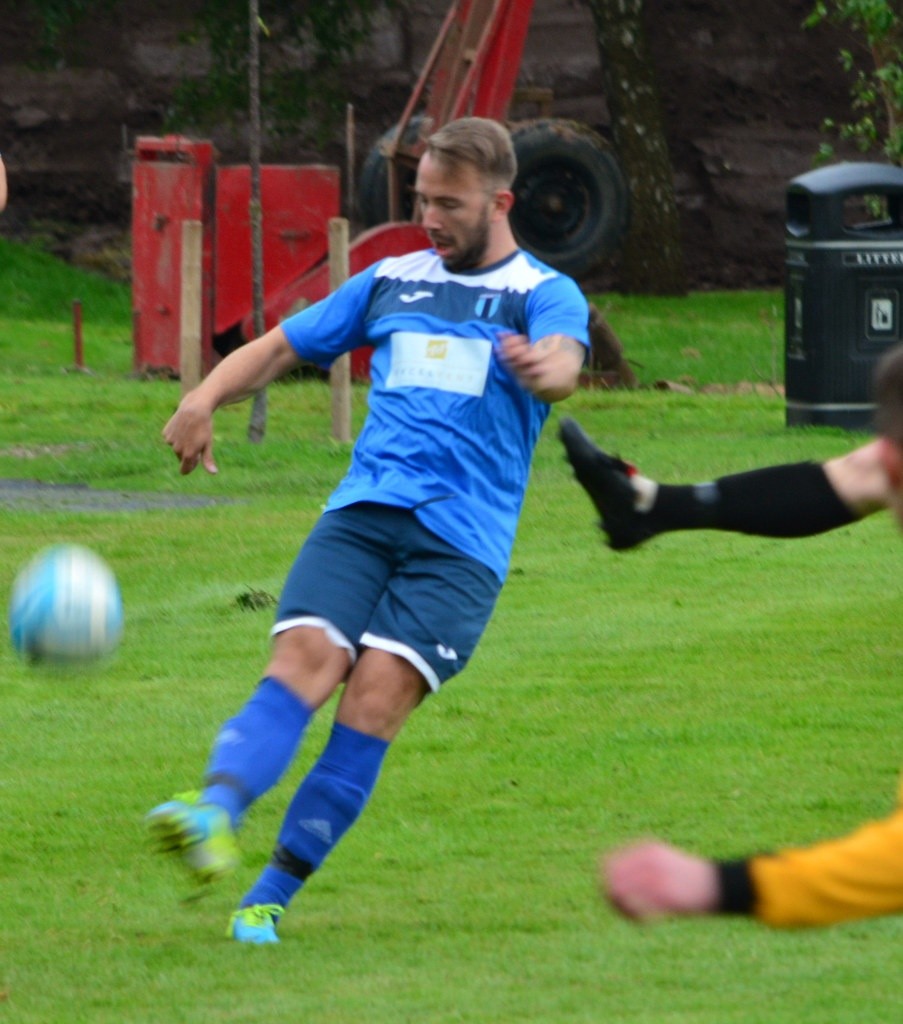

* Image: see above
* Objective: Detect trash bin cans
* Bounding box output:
[779,158,902,426]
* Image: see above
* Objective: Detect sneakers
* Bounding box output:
[148,792,236,883]
[227,904,285,949]
[559,416,654,552]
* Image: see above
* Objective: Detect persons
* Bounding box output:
[559,418,888,551]
[152,118,589,944]
[590,349,903,930]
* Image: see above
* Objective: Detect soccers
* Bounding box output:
[10,545,125,670]
